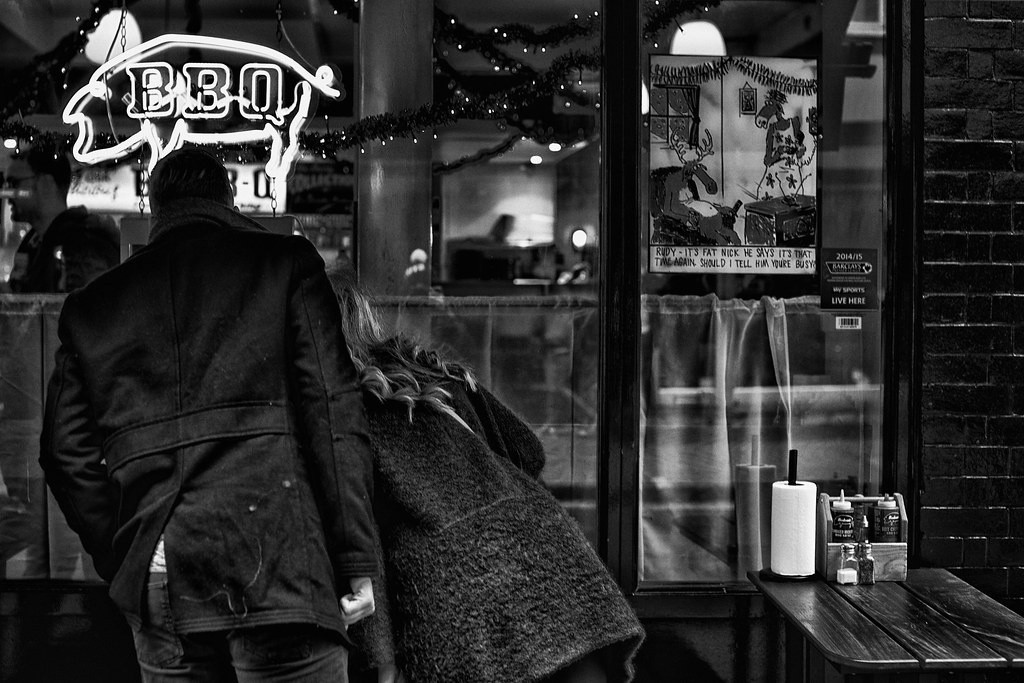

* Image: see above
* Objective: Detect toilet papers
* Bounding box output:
[733,463,777,572]
[770,480,817,576]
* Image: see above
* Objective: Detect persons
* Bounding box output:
[327,272,644,683]
[41,148,379,683]
[489,215,518,244]
[4,143,115,291]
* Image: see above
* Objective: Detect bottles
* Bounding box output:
[836,543,876,586]
[875,493,899,542]
[831,488,854,541]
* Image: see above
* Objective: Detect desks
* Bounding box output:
[747,569,1024,683]
